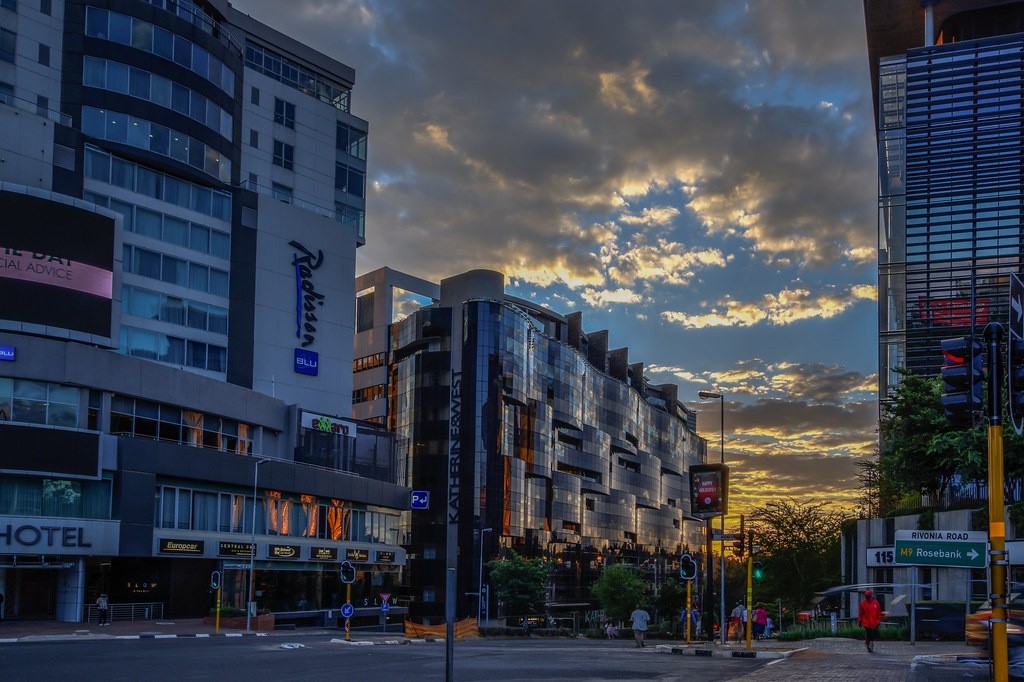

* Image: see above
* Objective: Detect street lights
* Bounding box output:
[698,390,724,644]
[477,528,493,628]
[246,458,270,634]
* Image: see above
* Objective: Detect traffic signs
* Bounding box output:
[893,531,989,570]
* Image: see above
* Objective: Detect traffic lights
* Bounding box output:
[679,555,696,580]
[939,336,983,424]
[340,561,355,584]
[753,562,762,582]
[211,571,221,589]
[1008,332,1023,435]
[733,534,744,556]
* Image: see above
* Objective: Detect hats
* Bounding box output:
[863,591,872,596]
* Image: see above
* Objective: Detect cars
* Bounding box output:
[964,592,1024,644]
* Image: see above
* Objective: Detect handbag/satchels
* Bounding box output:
[752,615,758,622]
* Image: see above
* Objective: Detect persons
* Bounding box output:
[858,591,882,653]
[680,603,701,641]
[96,593,111,626]
[630,604,650,648]
[731,600,774,642]
[606,619,615,640]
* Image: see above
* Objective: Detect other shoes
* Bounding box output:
[99,624,103,627]
[104,623,110,626]
